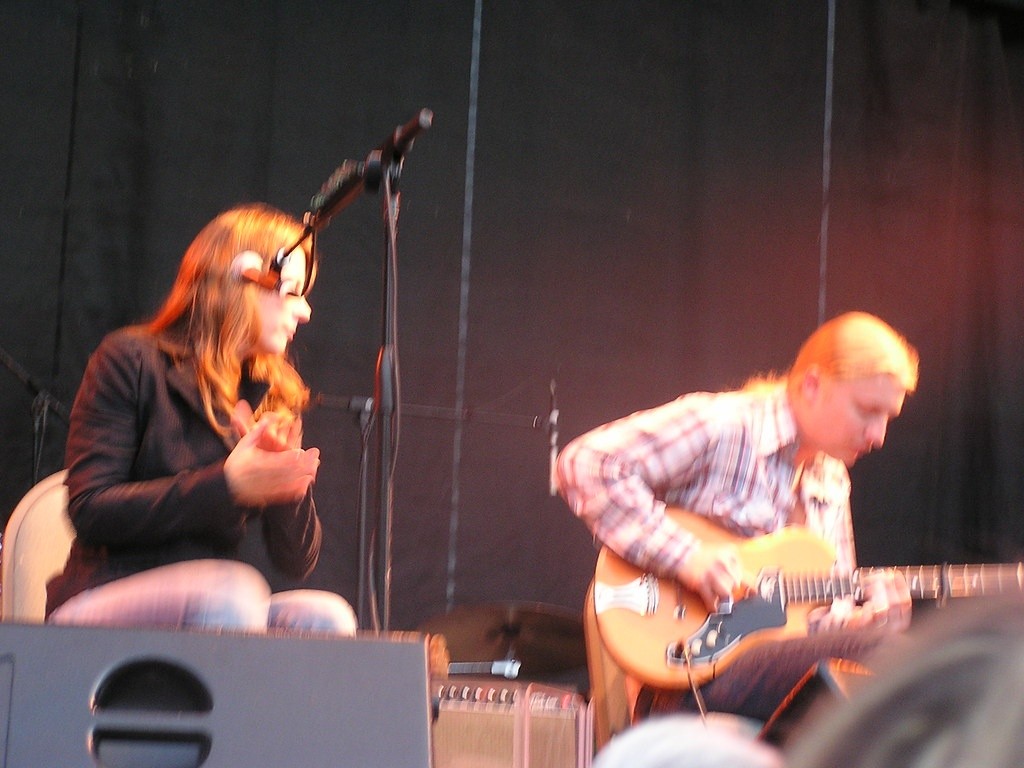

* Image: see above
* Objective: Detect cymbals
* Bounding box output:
[412,597,591,679]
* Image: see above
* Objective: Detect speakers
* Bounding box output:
[1,617,589,768]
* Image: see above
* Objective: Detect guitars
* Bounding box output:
[593,506,1024,689]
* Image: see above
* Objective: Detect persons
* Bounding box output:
[556,310,920,728]
[43,203,358,634]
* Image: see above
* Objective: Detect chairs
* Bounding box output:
[581,575,647,753]
[3,470,76,623]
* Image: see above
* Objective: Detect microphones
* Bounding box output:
[229,251,302,297]
